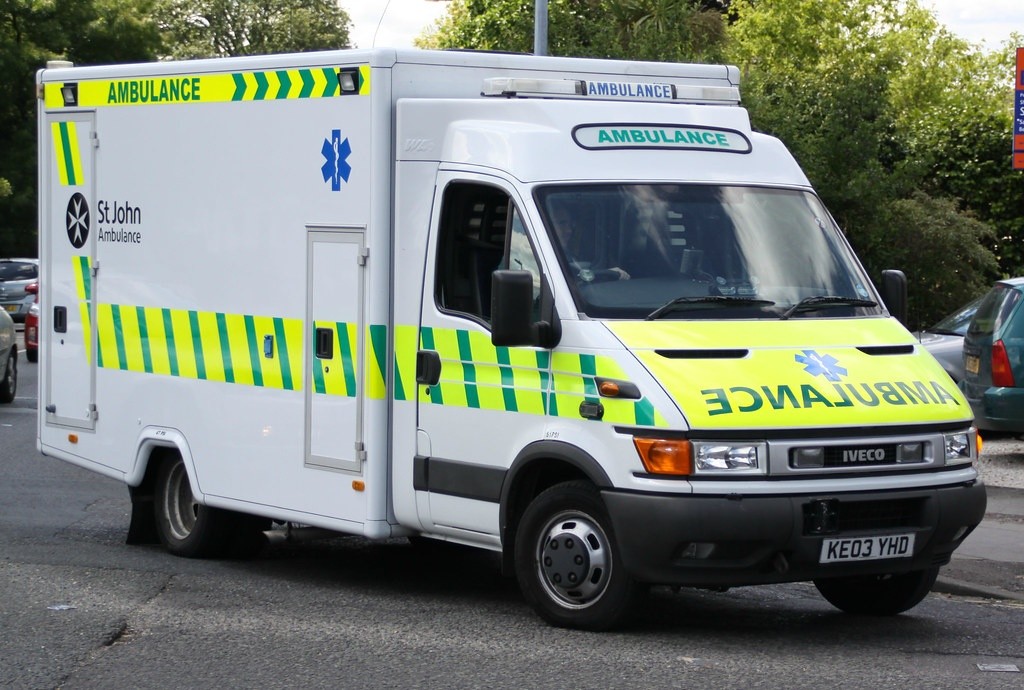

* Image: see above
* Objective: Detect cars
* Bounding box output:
[0,257,41,318]
[23,278,38,362]
[0,306,17,403]
[962,276,1024,435]
[907,289,987,383]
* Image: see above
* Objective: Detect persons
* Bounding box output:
[497,197,631,302]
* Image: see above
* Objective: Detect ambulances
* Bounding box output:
[36,42,989,629]
